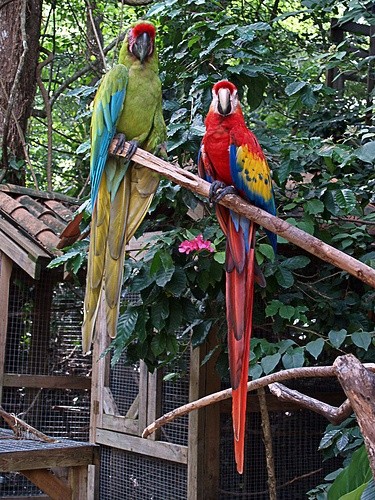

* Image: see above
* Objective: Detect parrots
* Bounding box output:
[79,20,169,357]
[197,78,276,476]
